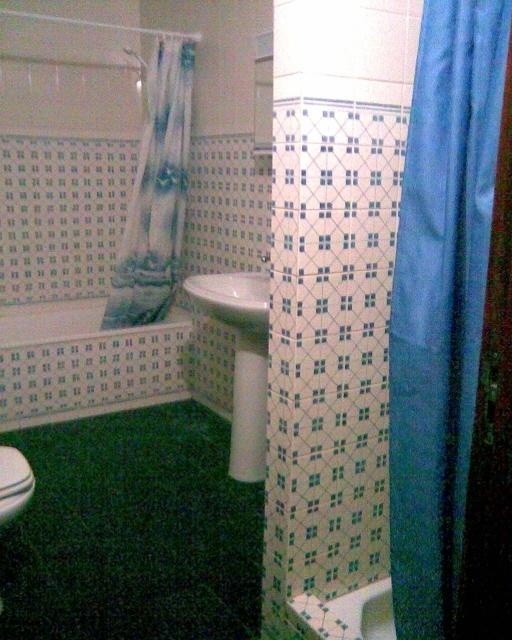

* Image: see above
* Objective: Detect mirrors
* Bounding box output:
[253,54,273,150]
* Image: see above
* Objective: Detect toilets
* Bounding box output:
[1,440,38,526]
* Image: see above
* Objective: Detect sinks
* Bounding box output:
[190,271,270,306]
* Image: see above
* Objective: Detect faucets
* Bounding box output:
[259,252,268,264]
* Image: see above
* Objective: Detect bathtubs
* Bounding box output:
[325,577,398,640]
[0,299,199,436]
